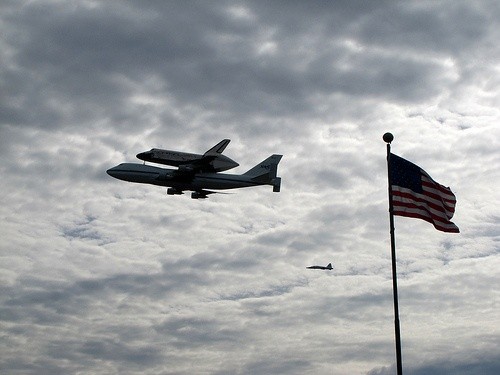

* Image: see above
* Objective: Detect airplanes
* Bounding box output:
[106,154,282,199]
[136,139,240,172]
[307,263,333,271]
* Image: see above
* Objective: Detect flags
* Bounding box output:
[388,152,460,233]
